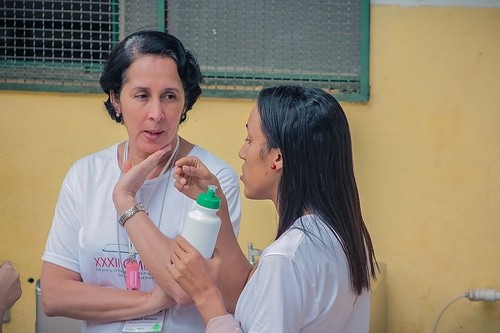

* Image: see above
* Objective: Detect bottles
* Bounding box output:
[174,185,222,260]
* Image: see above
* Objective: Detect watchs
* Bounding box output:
[117,202,150,227]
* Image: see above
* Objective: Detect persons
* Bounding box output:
[0,260,23,333]
[41,31,241,333]
[165,85,370,333]
